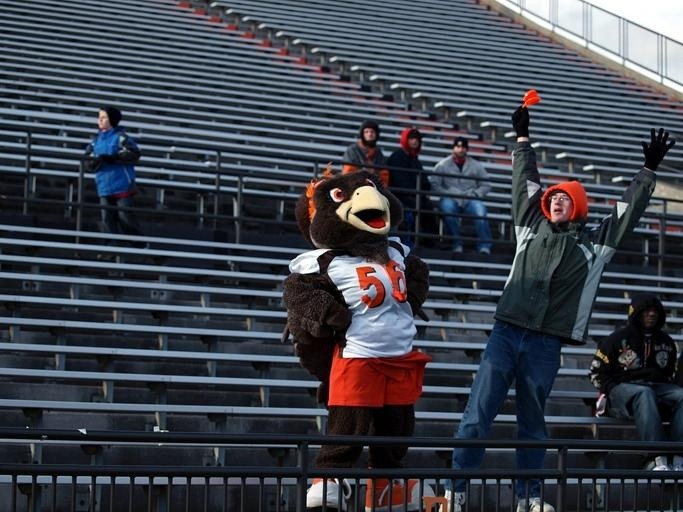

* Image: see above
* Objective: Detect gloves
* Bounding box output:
[511,104,530,139]
[99,153,119,164]
[641,128,676,171]
[86,161,101,173]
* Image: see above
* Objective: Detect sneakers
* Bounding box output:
[452,244,463,252]
[668,455,682,471]
[141,247,165,264]
[95,253,118,260]
[514,496,554,512]
[438,487,466,512]
[478,248,492,255]
[650,455,672,471]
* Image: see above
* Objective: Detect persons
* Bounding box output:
[79,103,141,232]
[382,127,439,252]
[437,89,677,512]
[338,119,388,185]
[587,289,683,474]
[416,134,494,259]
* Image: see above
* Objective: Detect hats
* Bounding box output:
[359,120,379,147]
[452,137,468,151]
[403,129,424,139]
[100,106,123,127]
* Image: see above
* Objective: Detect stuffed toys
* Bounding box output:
[276,168,442,512]
